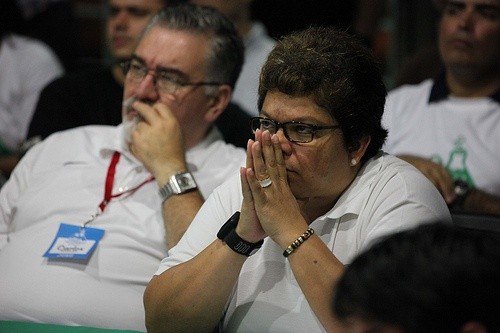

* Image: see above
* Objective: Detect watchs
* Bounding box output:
[448,180,469,210]
[217,210,264,258]
[159,170,197,205]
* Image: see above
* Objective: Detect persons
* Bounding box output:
[380,0,499,219]
[0,3,250,333]
[143,25,452,333]
[330,215,500,333]
[23,0,256,164]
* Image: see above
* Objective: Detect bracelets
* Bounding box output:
[283,226,315,258]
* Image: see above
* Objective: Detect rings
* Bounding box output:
[258,177,273,189]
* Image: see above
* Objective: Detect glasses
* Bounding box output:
[120,61,223,94]
[250,116,344,143]
